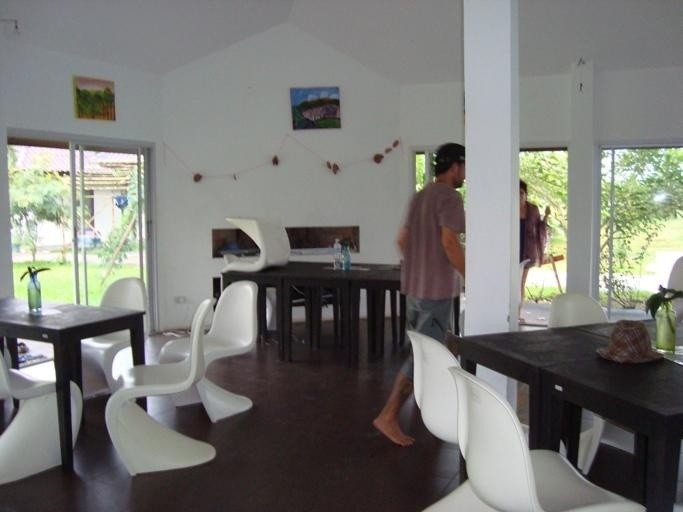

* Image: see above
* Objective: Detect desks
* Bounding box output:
[222,261,460,368]
[459,317,683,511]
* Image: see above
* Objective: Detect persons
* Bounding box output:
[519,177,546,327]
[371,142,466,447]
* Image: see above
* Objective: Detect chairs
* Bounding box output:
[405,329,567,511]
[667,256,683,318]
[549,291,609,475]
[222,215,291,272]
[459,259,532,336]
[422,368,647,512]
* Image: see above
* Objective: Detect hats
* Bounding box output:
[435,142,465,163]
[595,319,666,366]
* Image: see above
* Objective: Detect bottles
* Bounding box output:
[331,238,351,271]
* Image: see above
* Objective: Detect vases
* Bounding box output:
[28,274,41,312]
[343,245,351,270]
